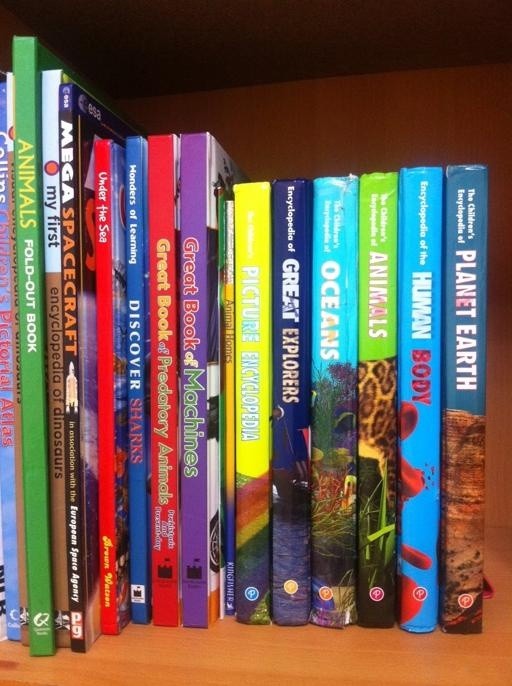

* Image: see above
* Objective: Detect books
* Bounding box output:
[1,36,489,656]
